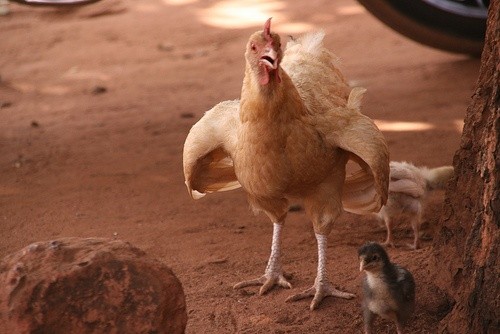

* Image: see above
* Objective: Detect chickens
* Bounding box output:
[356,241,416,334]
[182,17,390,311]
[372,158,455,247]
